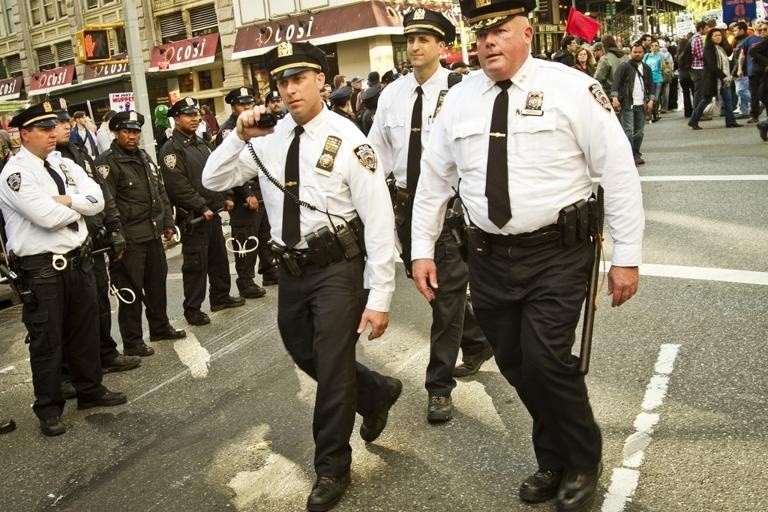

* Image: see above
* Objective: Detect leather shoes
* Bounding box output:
[427,393,454,423]
[756,123,768,141]
[41,415,66,436]
[101,356,141,374]
[746,117,757,123]
[451,341,494,377]
[727,123,743,127]
[186,310,211,325]
[307,467,352,510]
[517,466,560,501]
[150,327,185,341]
[210,295,246,312]
[688,122,702,131]
[122,343,154,356]
[735,114,749,119]
[634,156,644,164]
[359,378,403,440]
[262,271,279,285]
[77,389,127,408]
[555,457,602,509]
[239,283,265,298]
[60,380,77,399]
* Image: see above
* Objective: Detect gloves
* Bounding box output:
[109,233,127,255]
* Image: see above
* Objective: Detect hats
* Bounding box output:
[402,9,455,43]
[329,88,352,98]
[381,70,395,82]
[362,88,379,101]
[352,76,364,83]
[53,108,70,121]
[225,88,255,103]
[109,112,144,131]
[167,97,199,117]
[8,99,56,127]
[266,91,282,101]
[460,0,536,32]
[264,42,328,79]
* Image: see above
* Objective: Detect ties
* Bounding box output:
[485,78,514,227]
[282,126,305,249]
[406,87,423,200]
[43,160,78,232]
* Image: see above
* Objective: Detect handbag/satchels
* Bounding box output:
[628,61,654,120]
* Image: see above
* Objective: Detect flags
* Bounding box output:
[567,6,603,44]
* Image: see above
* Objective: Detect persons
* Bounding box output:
[750,35,768,140]
[92,111,187,355]
[201,41,403,510]
[641,35,652,51]
[70,112,98,161]
[321,69,401,136]
[366,10,495,422]
[551,36,577,67]
[730,22,738,32]
[675,39,696,117]
[592,41,604,60]
[155,105,173,146]
[410,0,646,510]
[1,416,17,438]
[214,87,280,299]
[569,49,592,75]
[201,105,221,143]
[737,19,765,124]
[593,35,629,106]
[690,21,714,121]
[158,97,247,325]
[643,40,669,123]
[48,96,141,398]
[96,110,118,155]
[662,37,679,109]
[759,22,768,36]
[612,45,655,166]
[703,19,716,44]
[715,23,735,45]
[729,22,764,119]
[265,88,286,118]
[195,109,212,142]
[1,101,128,436]
[656,39,674,114]
[738,18,755,36]
[439,58,482,74]
[688,29,744,130]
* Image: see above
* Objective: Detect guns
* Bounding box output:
[1,265,37,310]
[446,196,468,262]
[397,190,412,224]
[268,242,304,280]
[179,208,191,233]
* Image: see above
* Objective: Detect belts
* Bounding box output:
[268,227,363,265]
[15,242,93,280]
[475,225,564,247]
